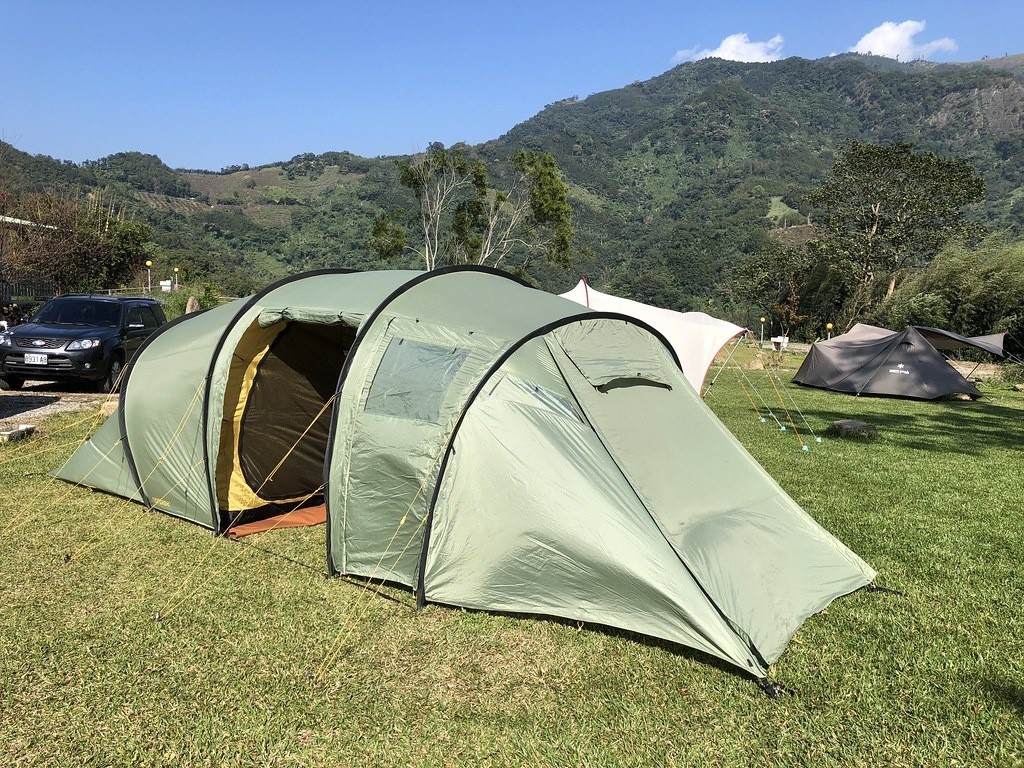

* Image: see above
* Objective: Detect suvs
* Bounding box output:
[0,294,167,394]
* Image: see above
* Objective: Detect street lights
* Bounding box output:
[174,268,178,287]
[760,317,765,343]
[827,323,832,340]
[146,261,152,292]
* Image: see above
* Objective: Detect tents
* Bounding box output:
[46,263,881,697]
[792,322,1008,402]
[556,275,749,398]
